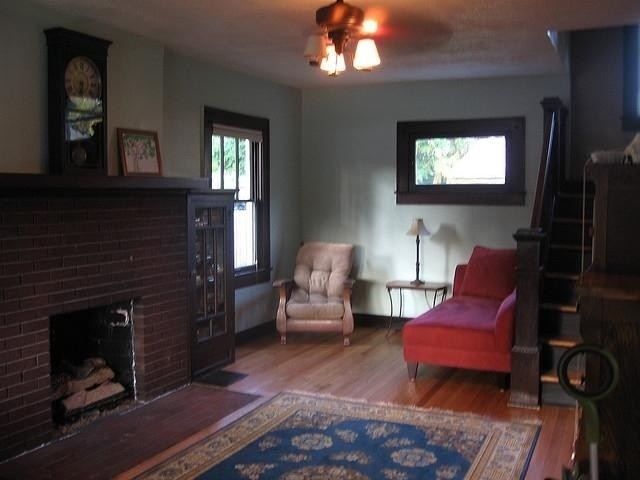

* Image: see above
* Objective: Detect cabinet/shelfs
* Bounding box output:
[188,189,239,376]
[560,158,639,479]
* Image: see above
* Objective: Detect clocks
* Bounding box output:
[42,24,112,178]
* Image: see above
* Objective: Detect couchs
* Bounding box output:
[401,264,518,390]
[271,239,357,346]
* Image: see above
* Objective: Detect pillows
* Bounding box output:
[460,243,519,299]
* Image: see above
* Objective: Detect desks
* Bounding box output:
[385,280,450,340]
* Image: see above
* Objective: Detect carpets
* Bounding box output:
[193,368,248,387]
[129,391,545,479]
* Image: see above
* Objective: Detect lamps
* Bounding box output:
[404,216,431,282]
[302,0,386,76]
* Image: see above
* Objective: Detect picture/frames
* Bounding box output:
[115,127,164,177]
